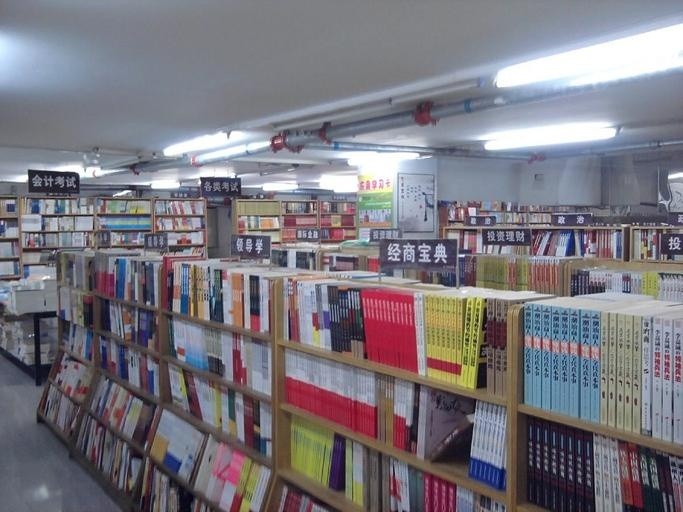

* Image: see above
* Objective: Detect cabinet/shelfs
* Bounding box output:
[0,196,681,511]
